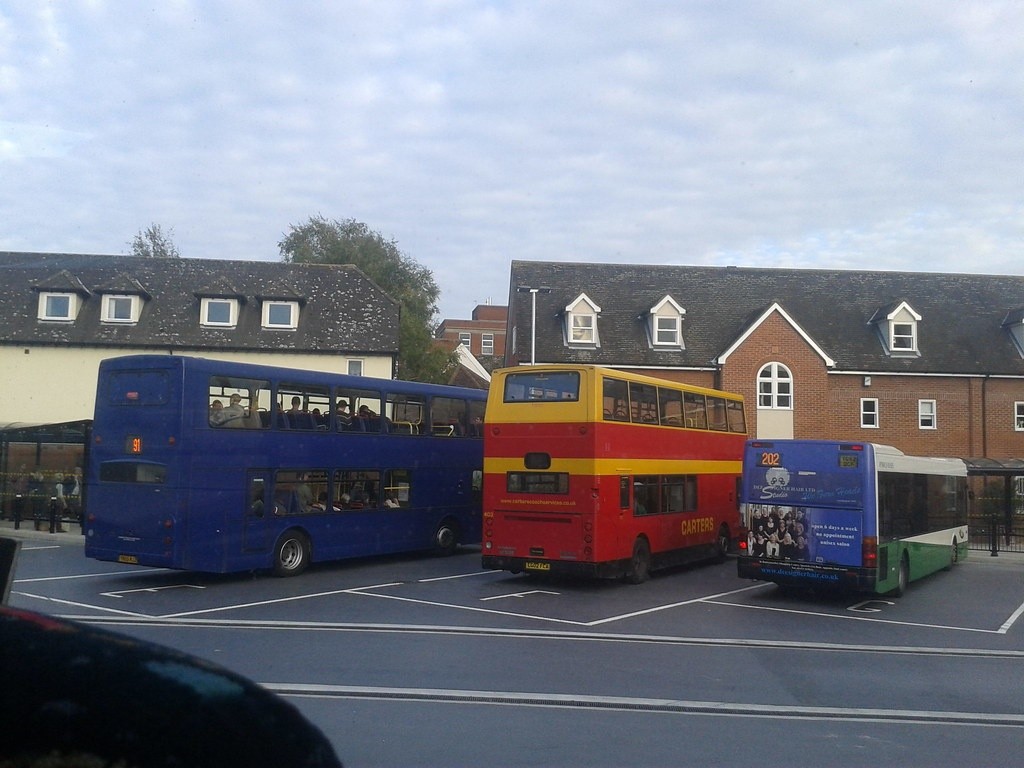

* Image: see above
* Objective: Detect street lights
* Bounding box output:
[517,285,552,365]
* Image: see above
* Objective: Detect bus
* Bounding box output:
[738,440,976,598]
[80,355,489,578]
[481,364,750,585]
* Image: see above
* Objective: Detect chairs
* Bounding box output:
[602,408,682,426]
[311,501,364,511]
[241,405,420,435]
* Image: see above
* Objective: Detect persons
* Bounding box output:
[287,396,302,414]
[384,492,400,508]
[336,400,352,431]
[360,405,376,418]
[209,394,244,426]
[313,408,320,415]
[14,463,82,534]
[312,492,340,511]
[748,507,810,561]
